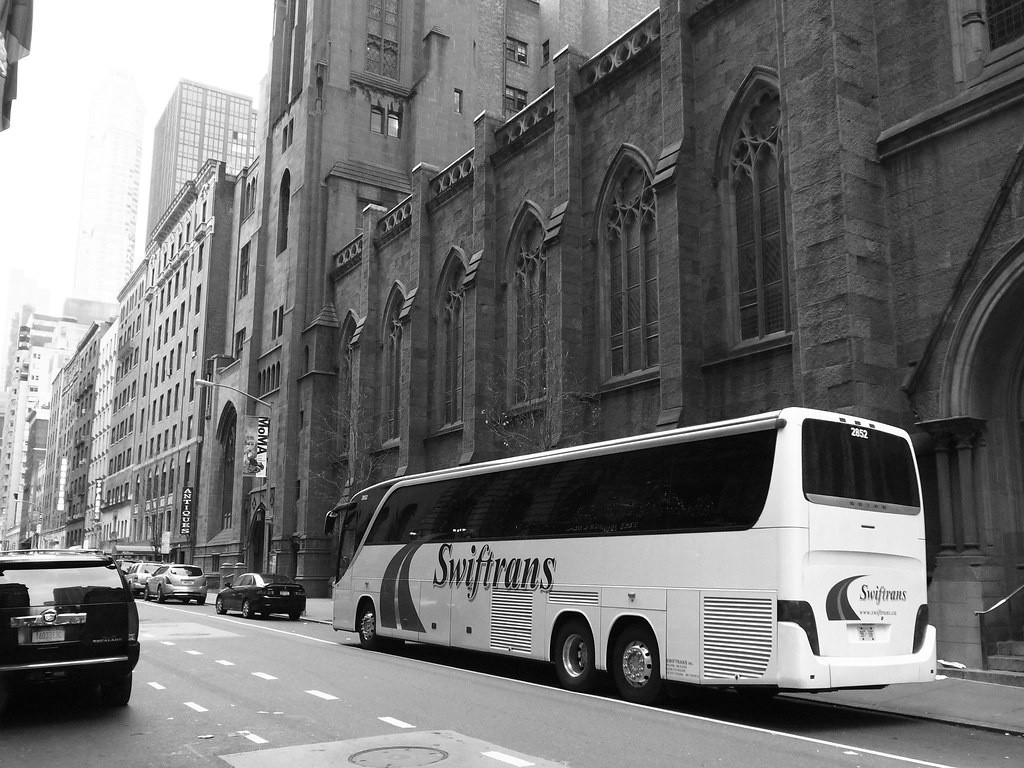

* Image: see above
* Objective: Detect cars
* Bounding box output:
[214,572,307,621]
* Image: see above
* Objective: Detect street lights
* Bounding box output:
[195,378,275,574]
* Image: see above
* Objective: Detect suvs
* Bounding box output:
[121,562,163,596]
[0,547,141,708]
[144,565,207,605]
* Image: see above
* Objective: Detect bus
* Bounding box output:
[325,407,940,708]
